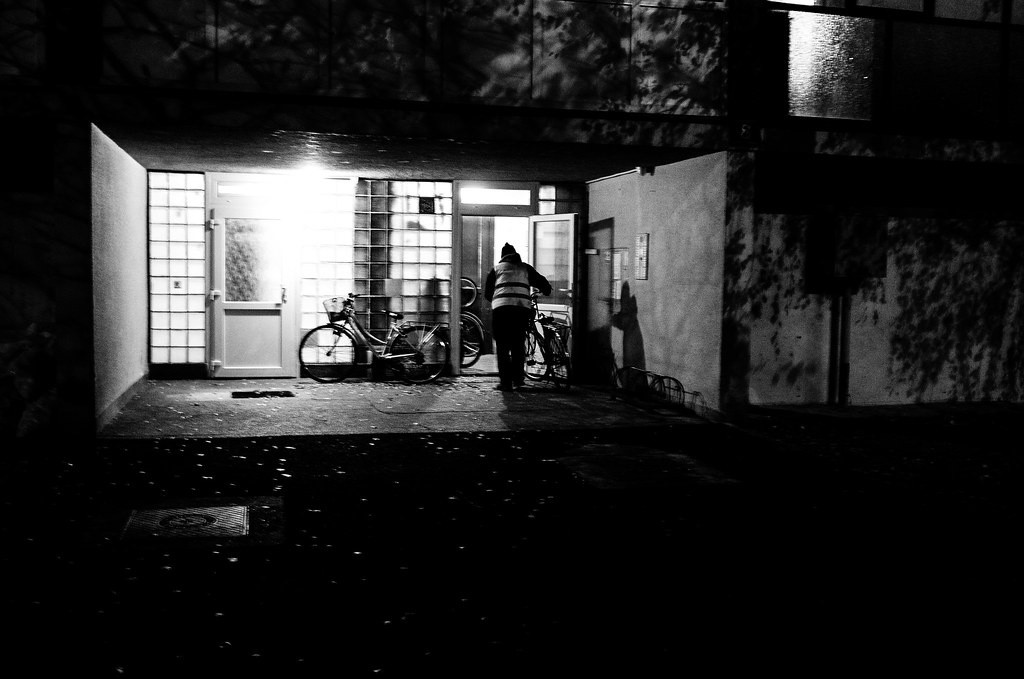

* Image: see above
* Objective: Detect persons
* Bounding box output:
[484,242,553,392]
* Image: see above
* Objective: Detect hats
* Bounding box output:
[501,242,516,258]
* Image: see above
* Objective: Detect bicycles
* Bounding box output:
[299,292,449,385]
[455,277,486,368]
[519,288,574,395]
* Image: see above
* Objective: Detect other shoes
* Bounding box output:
[495,383,512,392]
[513,379,526,387]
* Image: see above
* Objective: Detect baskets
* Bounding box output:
[323,297,346,322]
